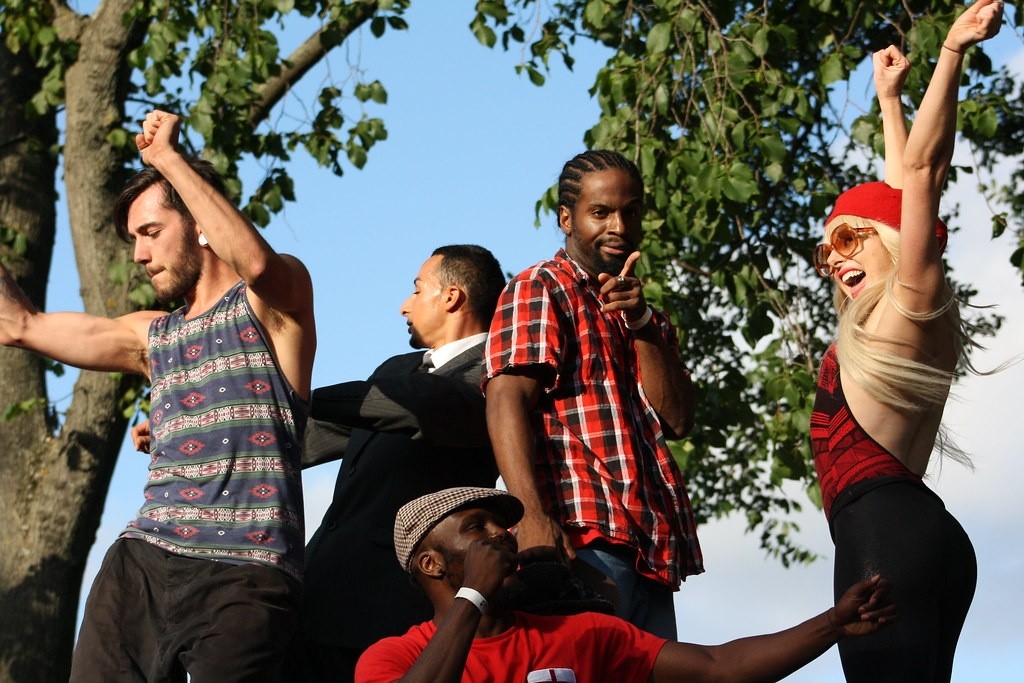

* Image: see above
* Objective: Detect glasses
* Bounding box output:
[812,223,878,278]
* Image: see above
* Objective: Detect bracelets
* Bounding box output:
[454,586,488,616]
[942,44,965,56]
[620,305,653,331]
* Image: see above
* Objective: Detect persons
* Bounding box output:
[354,486,900,683]
[810,0,1004,683]
[480,149,696,642]
[132,244,507,683]
[0,111,318,683]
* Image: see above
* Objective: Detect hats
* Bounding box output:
[824,182,947,258]
[394,487,524,575]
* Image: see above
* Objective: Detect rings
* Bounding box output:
[618,274,625,290]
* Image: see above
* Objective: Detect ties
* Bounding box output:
[416,353,435,374]
